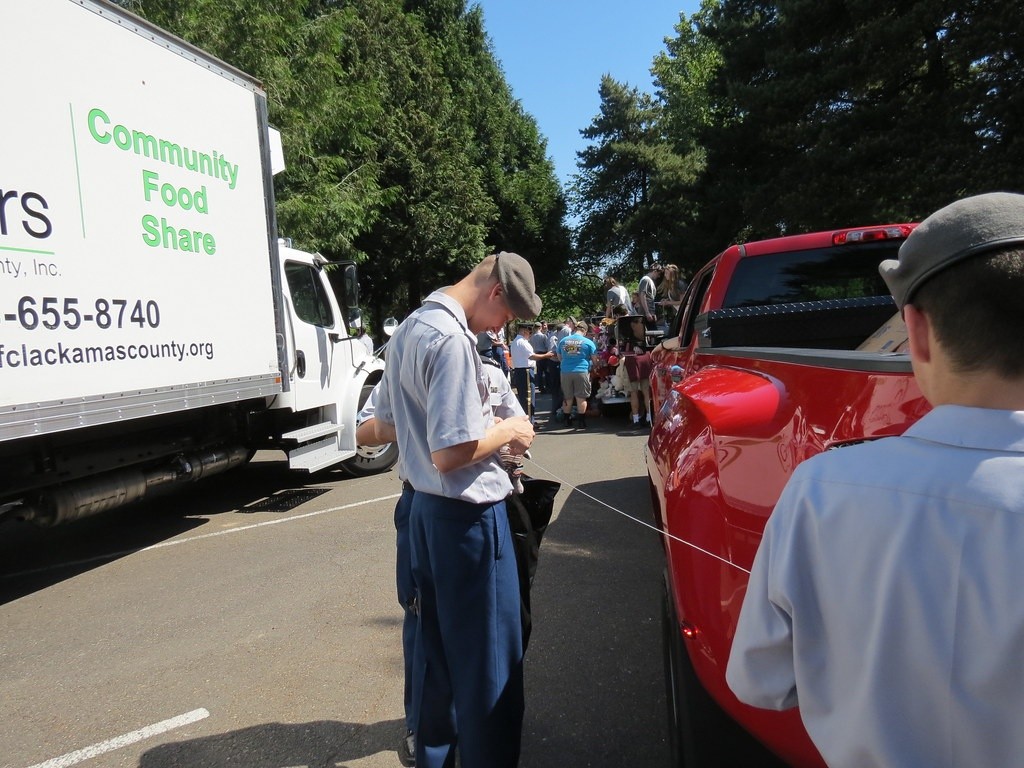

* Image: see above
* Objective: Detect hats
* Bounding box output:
[878,192,1024,320]
[569,315,588,332]
[517,320,566,332]
[498,250,542,320]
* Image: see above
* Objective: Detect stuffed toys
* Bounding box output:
[589,320,628,398]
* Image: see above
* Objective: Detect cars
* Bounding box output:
[614,219,939,767]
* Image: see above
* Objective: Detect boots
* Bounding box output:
[563,412,589,431]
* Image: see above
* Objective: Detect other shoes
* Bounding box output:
[633,420,651,430]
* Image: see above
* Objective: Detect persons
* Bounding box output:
[650,335,679,365]
[511,322,554,428]
[530,324,551,397]
[627,317,647,354]
[657,264,683,334]
[603,276,636,319]
[638,263,662,344]
[539,319,557,337]
[358,353,534,749]
[490,327,508,379]
[548,324,562,419]
[731,193,1023,768]
[559,314,578,354]
[372,252,543,768]
[612,302,629,339]
[475,323,498,365]
[630,290,640,310]
[557,321,600,427]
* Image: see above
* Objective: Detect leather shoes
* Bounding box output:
[402,727,416,766]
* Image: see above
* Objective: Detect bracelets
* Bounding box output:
[661,341,671,351]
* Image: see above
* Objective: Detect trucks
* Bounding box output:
[0,0,400,533]
